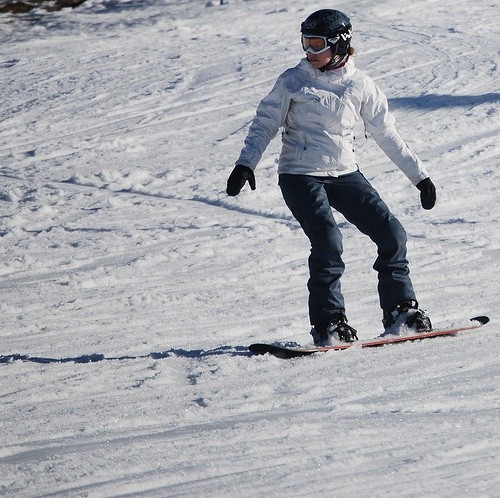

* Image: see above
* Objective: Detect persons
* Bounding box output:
[225,7,436,345]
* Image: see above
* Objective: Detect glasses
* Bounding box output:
[301,34,330,54]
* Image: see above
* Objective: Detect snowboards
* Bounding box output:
[250,315,490,354]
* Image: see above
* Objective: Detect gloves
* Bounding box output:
[416,177,436,210]
[226,165,256,196]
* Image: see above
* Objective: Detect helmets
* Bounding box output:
[301,9,352,56]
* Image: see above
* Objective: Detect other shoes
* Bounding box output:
[309,320,359,346]
[382,301,432,332]
